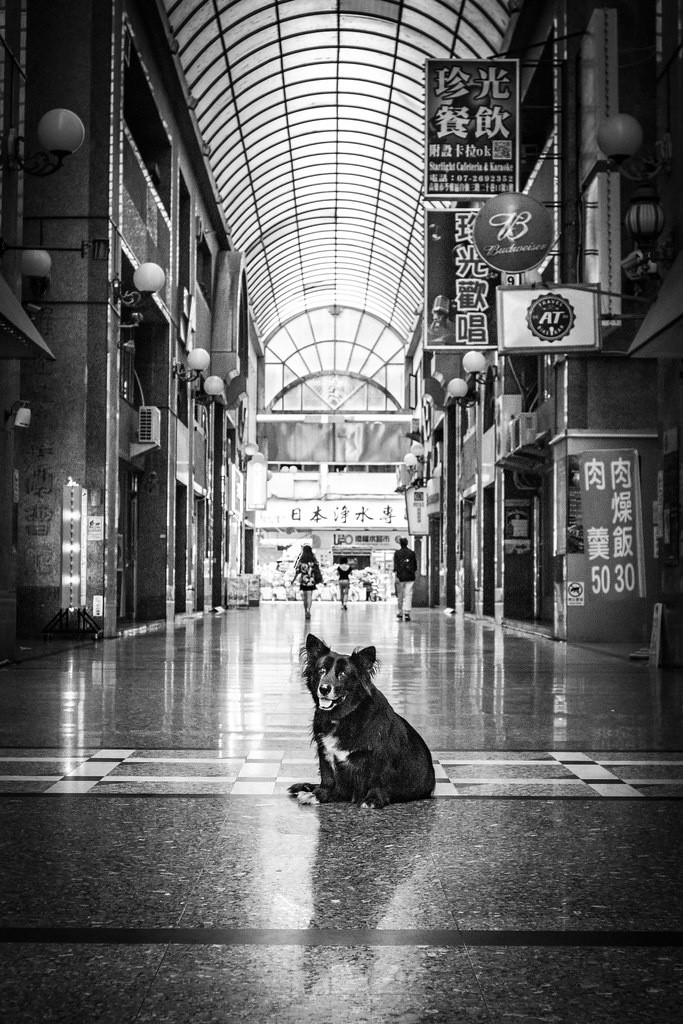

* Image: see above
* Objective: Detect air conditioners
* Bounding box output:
[494,395,522,461]
[138,405,162,449]
[507,411,537,451]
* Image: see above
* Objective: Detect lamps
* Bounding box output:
[403,443,432,490]
[462,350,497,386]
[448,378,481,410]
[110,262,166,305]
[241,442,260,471]
[174,348,211,383]
[0,107,86,179]
[4,400,34,428]
[0,234,111,260]
[596,111,671,180]
[20,248,49,302]
[194,375,224,407]
[627,180,677,268]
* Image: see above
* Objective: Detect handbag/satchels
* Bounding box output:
[314,562,322,583]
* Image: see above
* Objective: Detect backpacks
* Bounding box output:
[399,549,415,582]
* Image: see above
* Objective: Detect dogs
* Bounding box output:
[286,633,436,809]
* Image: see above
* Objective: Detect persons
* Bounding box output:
[394,538,417,620]
[291,546,323,618]
[336,557,352,610]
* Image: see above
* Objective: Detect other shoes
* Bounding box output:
[405,614,410,620]
[397,613,403,618]
[306,613,311,619]
